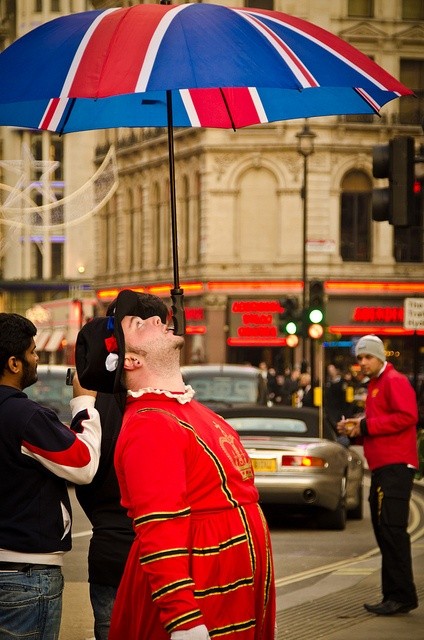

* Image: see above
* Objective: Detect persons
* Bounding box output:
[75,289,276,639]
[335,335,418,616]
[0,312,102,639]
[247,361,352,421]
[77,392,137,639]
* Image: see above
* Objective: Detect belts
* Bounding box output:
[1,561,61,571]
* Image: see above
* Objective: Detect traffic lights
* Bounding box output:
[308,280,324,339]
[371,137,414,226]
[285,320,300,347]
[278,299,294,332]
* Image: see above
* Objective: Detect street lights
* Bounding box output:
[295,124,316,310]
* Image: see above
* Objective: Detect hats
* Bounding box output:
[355,334,385,363]
[75,289,168,416]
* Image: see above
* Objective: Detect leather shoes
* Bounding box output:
[364,599,418,616]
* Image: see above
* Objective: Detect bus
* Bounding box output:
[23,365,267,422]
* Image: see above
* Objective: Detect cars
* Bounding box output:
[215,407,364,529]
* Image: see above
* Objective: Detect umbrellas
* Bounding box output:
[0,3,417,336]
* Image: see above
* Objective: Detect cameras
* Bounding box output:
[66,369,74,386]
